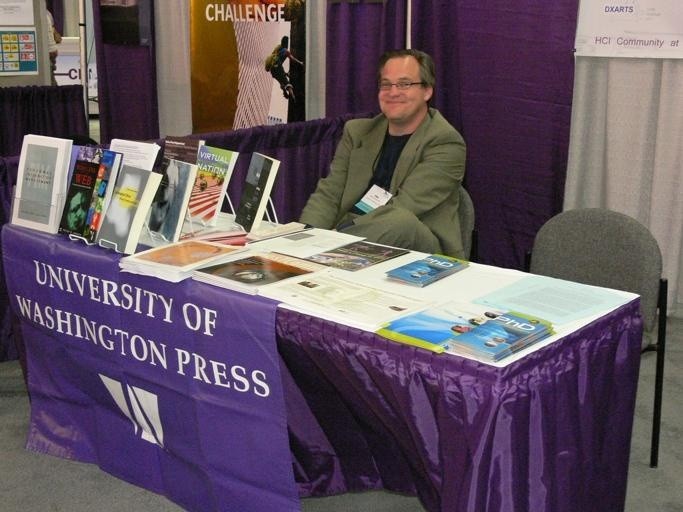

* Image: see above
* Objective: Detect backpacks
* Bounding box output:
[265,49,284,72]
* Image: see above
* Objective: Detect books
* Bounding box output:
[373,298,556,368]
[302,238,412,273]
[383,252,469,289]
[10,132,318,297]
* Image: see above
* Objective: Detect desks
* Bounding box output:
[0,211,643,511]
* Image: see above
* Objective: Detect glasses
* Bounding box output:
[377,81,423,90]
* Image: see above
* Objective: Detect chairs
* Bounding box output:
[520,208,670,468]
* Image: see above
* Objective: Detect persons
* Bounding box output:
[297,48,476,260]
[45,0,68,87]
[264,35,304,106]
